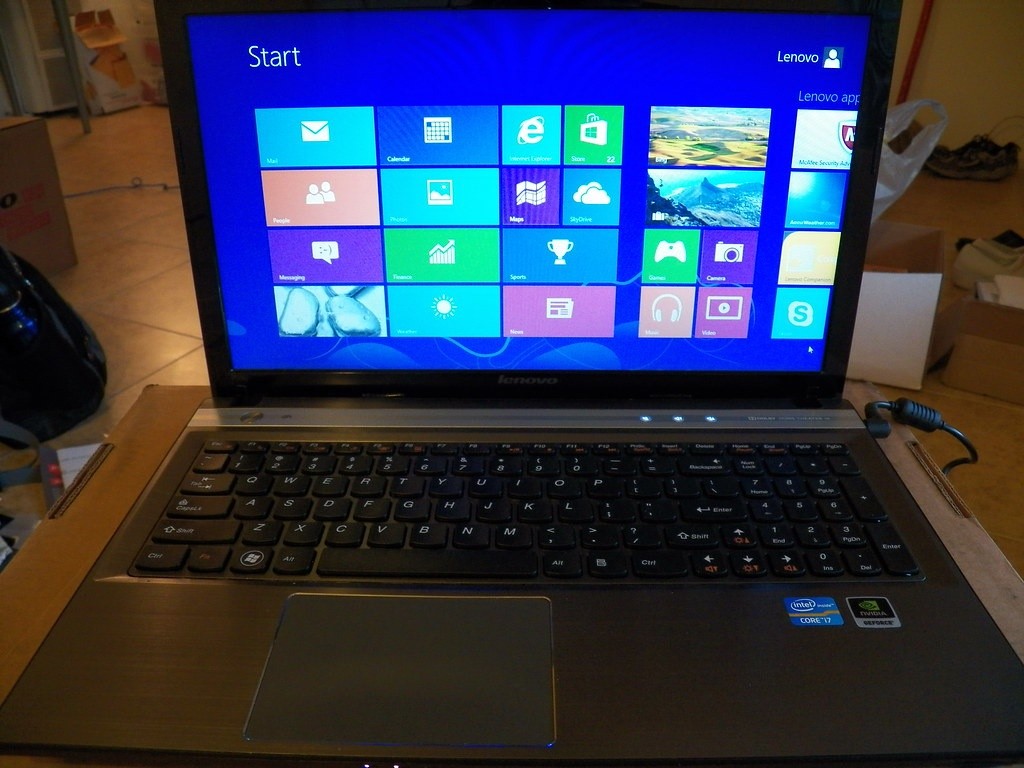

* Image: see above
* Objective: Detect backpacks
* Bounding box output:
[0,248,108,449]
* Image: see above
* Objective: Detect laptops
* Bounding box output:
[0,0,1024,768]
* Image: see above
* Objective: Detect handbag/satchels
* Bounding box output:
[870,98,949,226]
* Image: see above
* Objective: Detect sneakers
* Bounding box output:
[924,134,1020,181]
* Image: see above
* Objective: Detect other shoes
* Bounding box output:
[955,230,1024,252]
[950,238,1024,291]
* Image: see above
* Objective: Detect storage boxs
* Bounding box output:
[0,385,1024,768]
[0,115,79,275]
[71,8,144,115]
[926,298,1024,406]
[864,221,947,282]
